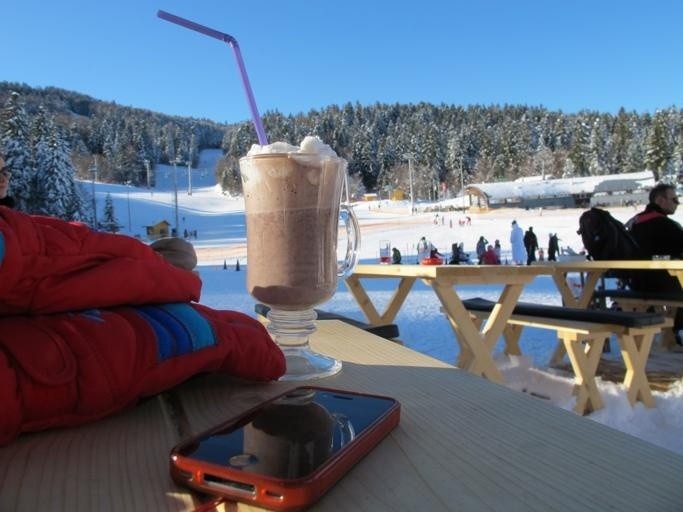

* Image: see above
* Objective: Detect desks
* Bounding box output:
[336,265,556,384]
[0,319,683,512]
[531,258,683,369]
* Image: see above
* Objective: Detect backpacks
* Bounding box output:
[576,207,638,278]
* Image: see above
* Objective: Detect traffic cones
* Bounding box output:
[223,259,227,269]
[236,258,241,271]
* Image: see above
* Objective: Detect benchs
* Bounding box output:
[439,297,624,416]
[255,302,403,345]
[602,309,674,409]
[591,289,683,350]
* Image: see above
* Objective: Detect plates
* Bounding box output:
[557,254,586,261]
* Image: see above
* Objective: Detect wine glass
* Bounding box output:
[238,152,361,381]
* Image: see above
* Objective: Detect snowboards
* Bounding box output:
[548,233,555,252]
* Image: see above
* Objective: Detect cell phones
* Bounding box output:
[167,383,401,512]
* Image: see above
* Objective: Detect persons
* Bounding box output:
[0,155,15,207]
[386,200,581,266]
[623,185,683,338]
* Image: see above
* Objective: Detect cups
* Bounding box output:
[417,240,431,265]
[380,240,392,265]
